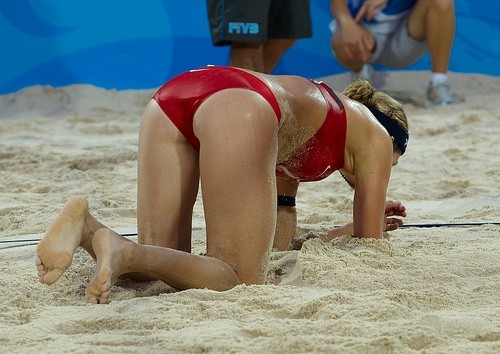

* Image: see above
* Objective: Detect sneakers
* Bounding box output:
[427,82,465,104]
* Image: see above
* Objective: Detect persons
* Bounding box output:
[35,64,411,305]
[204,0,315,77]
[327,0,468,107]
